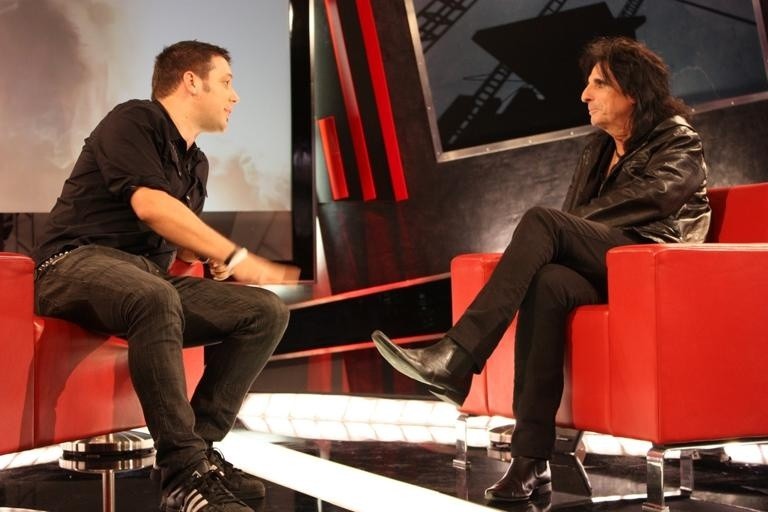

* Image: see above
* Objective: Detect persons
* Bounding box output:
[30,38,290,512]
[372,36,712,501]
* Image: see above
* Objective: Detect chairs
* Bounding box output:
[447,184,768,510]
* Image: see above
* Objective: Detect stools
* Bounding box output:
[0,249,207,456]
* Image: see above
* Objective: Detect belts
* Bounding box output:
[34,249,72,279]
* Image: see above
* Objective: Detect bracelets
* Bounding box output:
[224,243,244,265]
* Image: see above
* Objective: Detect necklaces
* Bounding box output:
[614,149,625,159]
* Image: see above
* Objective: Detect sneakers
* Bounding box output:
[149,448,265,501]
[157,458,254,512]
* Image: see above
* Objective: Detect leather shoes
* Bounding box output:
[483,457,552,502]
[370,329,474,408]
